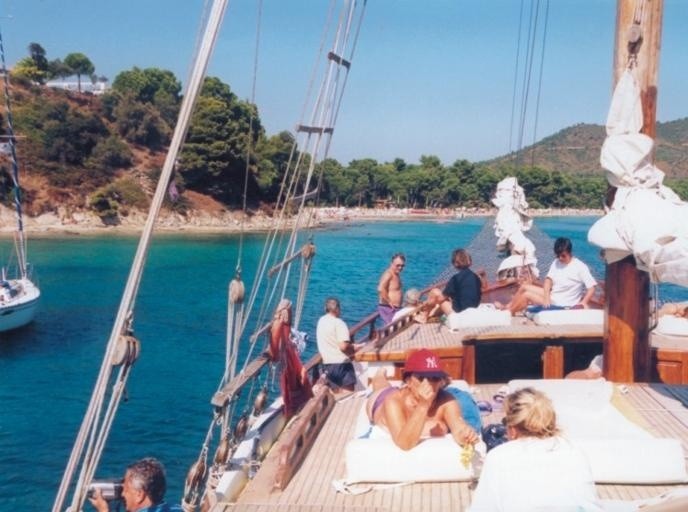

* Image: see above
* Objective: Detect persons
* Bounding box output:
[87,458,184,512]
[494,238,597,316]
[412,249,482,324]
[316,299,357,393]
[468,388,598,512]
[390,289,420,333]
[366,350,479,449]
[650,299,688,319]
[377,254,405,325]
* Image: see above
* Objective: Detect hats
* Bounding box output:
[402,350,448,383]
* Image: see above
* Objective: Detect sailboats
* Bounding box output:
[43,0,688,510]
[0,33,46,337]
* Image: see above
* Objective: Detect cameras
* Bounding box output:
[87,480,123,502]
